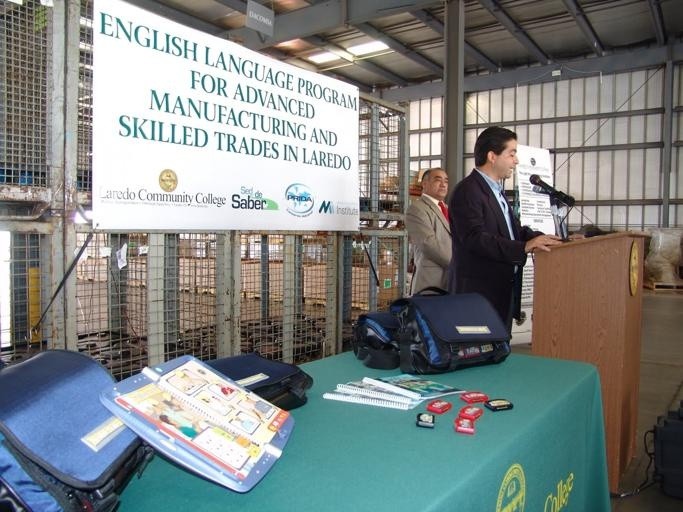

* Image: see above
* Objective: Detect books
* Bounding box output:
[323,390,416,410]
[363,373,466,399]
[337,382,420,404]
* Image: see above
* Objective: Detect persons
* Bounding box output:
[159,414,202,438]
[404,167,452,294]
[443,126,562,338]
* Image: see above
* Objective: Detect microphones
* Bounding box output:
[529,175,575,207]
[533,185,549,194]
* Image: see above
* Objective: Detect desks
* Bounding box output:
[115,350,613,511]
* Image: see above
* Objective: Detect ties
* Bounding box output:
[499,192,509,214]
[438,200,449,224]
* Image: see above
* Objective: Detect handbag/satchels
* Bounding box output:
[0,349,155,512]
[644,400,683,498]
[204,353,313,411]
[352,286,511,375]
